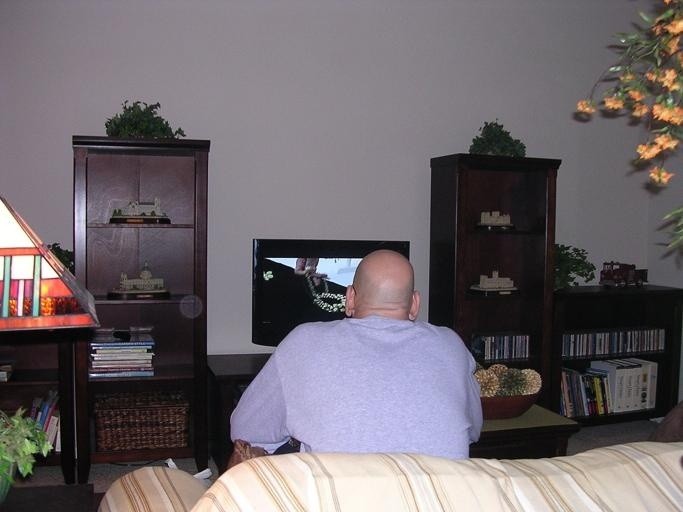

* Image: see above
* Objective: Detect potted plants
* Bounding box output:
[553,242,597,293]
[0,405,54,505]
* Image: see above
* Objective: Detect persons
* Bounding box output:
[229,249,485,459]
[294,258,324,286]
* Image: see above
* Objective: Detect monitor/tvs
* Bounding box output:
[252,238,410,347]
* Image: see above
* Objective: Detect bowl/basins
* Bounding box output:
[479,391,542,421]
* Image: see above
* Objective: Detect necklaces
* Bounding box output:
[305,270,348,314]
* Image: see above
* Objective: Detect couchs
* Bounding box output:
[95,441,683,512]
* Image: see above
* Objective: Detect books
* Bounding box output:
[476,332,530,361]
[29,389,61,454]
[87,328,157,378]
[559,326,666,419]
[0,360,17,383]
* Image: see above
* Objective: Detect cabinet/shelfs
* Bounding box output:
[0,329,96,485]
[70,134,212,484]
[552,284,682,427]
[428,153,562,412]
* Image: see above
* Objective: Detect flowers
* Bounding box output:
[575,0,683,186]
